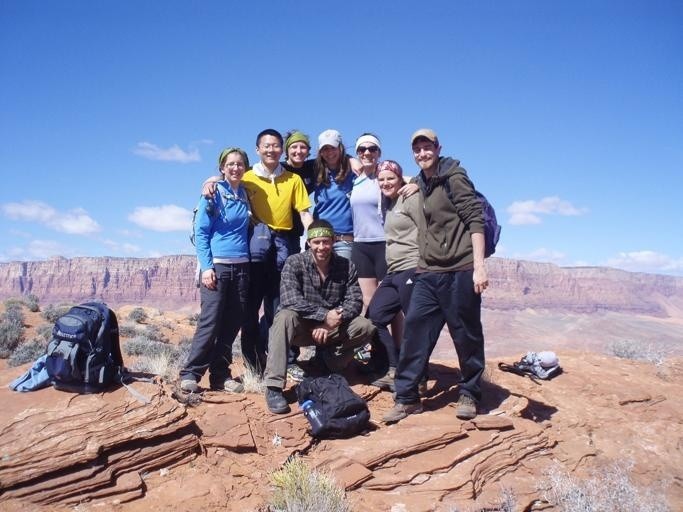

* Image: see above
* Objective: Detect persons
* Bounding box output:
[178,125,493,422]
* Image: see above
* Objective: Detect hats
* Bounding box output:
[411,128,438,146]
[318,129,341,151]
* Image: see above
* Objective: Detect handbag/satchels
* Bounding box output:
[444,174,501,259]
[514,353,561,379]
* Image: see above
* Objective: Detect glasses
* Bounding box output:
[225,162,245,170]
[357,145,378,154]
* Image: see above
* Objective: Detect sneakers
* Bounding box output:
[456,393,477,419]
[287,364,308,382]
[382,402,423,423]
[180,380,200,392]
[357,359,427,396]
[211,379,245,394]
[265,387,290,415]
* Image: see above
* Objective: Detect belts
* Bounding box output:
[336,234,353,241]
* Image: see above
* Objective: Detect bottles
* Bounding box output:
[301,401,324,435]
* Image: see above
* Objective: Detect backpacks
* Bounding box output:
[295,371,371,439]
[188,181,251,247]
[45,301,132,395]
